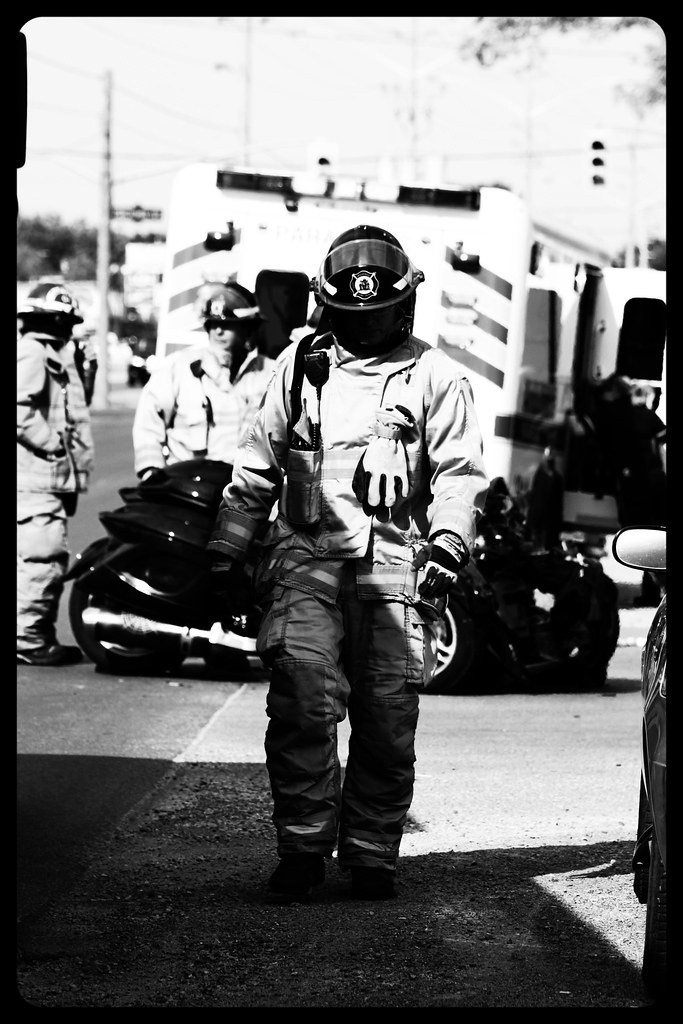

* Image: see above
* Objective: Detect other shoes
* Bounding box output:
[266,855,395,902]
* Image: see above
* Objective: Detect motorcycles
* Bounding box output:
[53,457,493,696]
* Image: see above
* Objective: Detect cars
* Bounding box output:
[610,526,683,1023]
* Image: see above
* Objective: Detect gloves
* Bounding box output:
[411,534,468,600]
[352,406,417,523]
[212,561,249,631]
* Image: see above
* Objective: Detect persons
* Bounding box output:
[17,282,96,667]
[130,280,288,523]
[204,224,491,905]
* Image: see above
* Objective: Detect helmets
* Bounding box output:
[17,282,84,323]
[312,224,424,358]
[202,286,259,325]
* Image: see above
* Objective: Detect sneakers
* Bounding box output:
[20,643,79,668]
